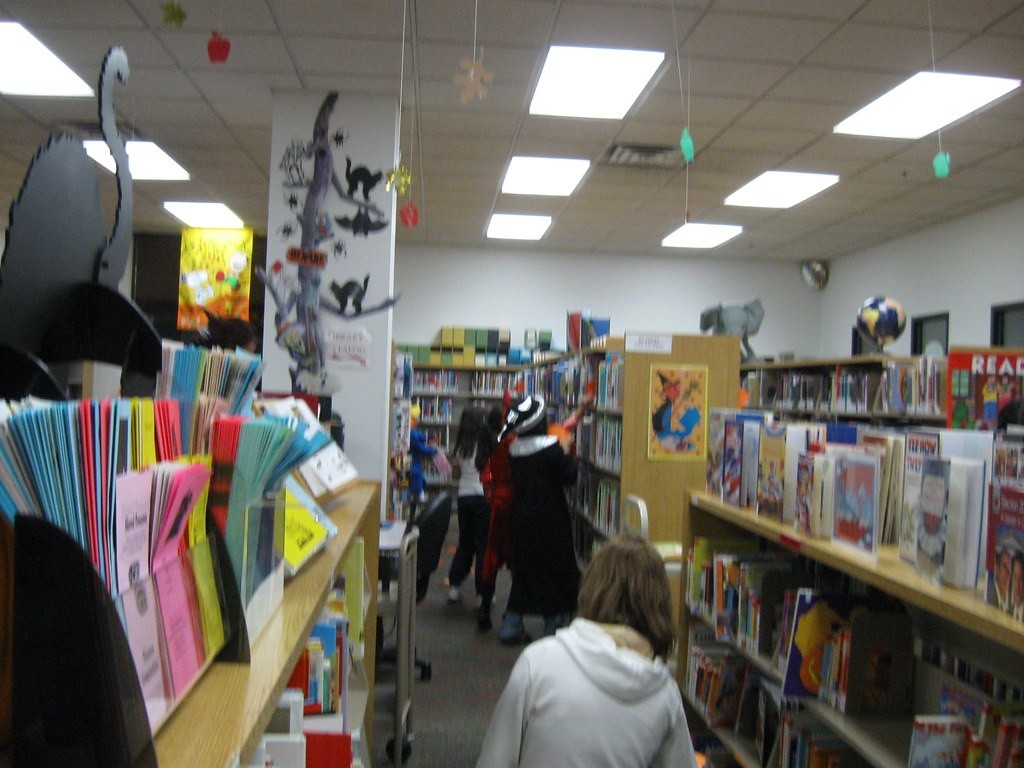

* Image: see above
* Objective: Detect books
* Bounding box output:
[393,360,1024,768]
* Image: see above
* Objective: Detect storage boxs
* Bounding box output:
[397,325,566,366]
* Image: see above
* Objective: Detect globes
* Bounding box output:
[857,294,909,356]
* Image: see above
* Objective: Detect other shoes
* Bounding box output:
[448,589,466,602]
[501,624,534,645]
[478,609,492,629]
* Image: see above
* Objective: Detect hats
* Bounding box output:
[495,396,548,444]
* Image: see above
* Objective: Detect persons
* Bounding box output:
[407,431,452,606]
[477,535,698,768]
[497,394,583,645]
[449,408,497,609]
[478,391,595,634]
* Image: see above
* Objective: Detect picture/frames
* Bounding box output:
[647,362,709,462]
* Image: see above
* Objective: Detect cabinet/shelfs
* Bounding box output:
[150,478,383,767]
[388,334,1024,768]
